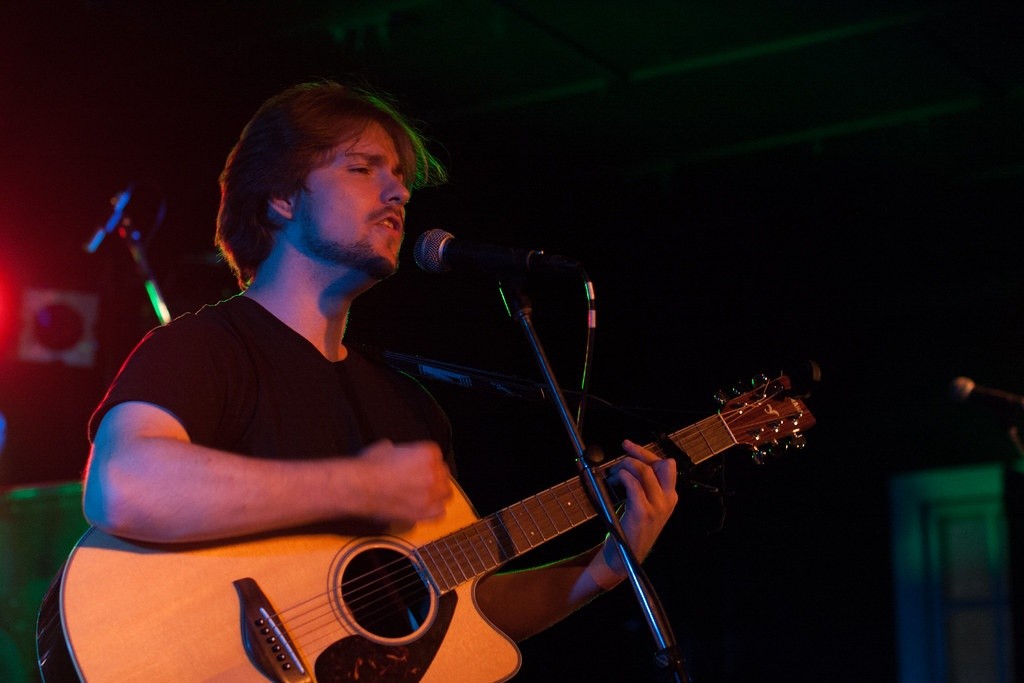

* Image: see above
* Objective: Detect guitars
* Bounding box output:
[29,368,824,683]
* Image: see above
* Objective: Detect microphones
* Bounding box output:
[950,376,1024,416]
[87,189,132,253]
[414,227,577,274]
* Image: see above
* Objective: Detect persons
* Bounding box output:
[83,82,678,645]
